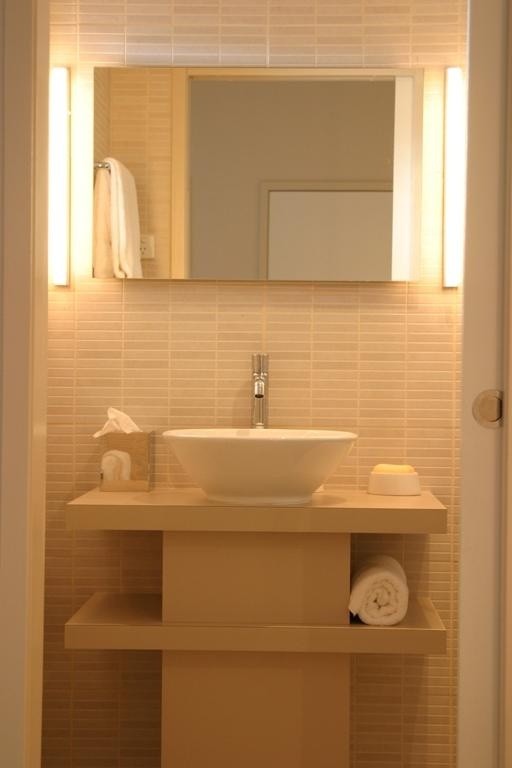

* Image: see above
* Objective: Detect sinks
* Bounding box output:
[162,428,360,504]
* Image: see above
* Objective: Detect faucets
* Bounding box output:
[251,351,269,429]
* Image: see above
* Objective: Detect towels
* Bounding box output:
[348,554,409,627]
[94,157,141,280]
[99,450,131,490]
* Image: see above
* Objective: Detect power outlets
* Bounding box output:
[137,235,154,259]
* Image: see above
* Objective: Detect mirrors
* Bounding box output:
[169,66,413,282]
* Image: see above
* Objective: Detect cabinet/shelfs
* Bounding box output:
[62,487,448,766]
